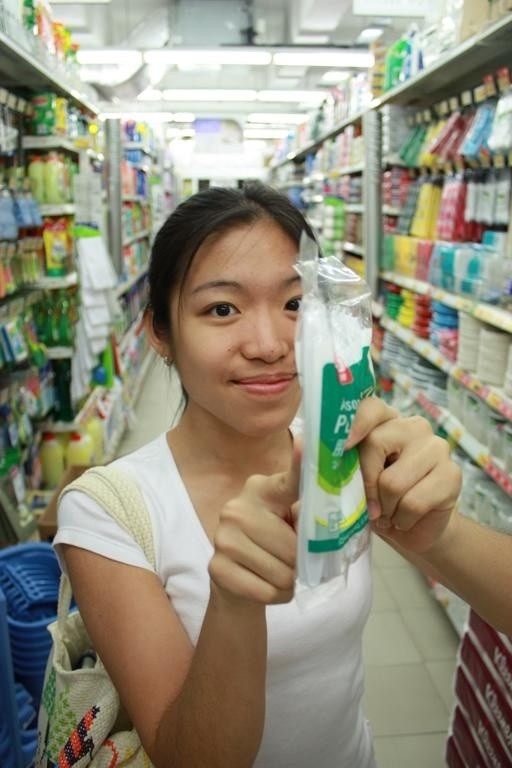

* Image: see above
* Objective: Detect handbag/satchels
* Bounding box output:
[35,610,154,768]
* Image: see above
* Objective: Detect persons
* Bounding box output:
[50,180,512,768]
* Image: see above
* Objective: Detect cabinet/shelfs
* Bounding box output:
[1,28,187,539]
[263,16,512,640]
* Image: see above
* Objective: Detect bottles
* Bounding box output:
[9,150,80,205]
[39,417,104,491]
